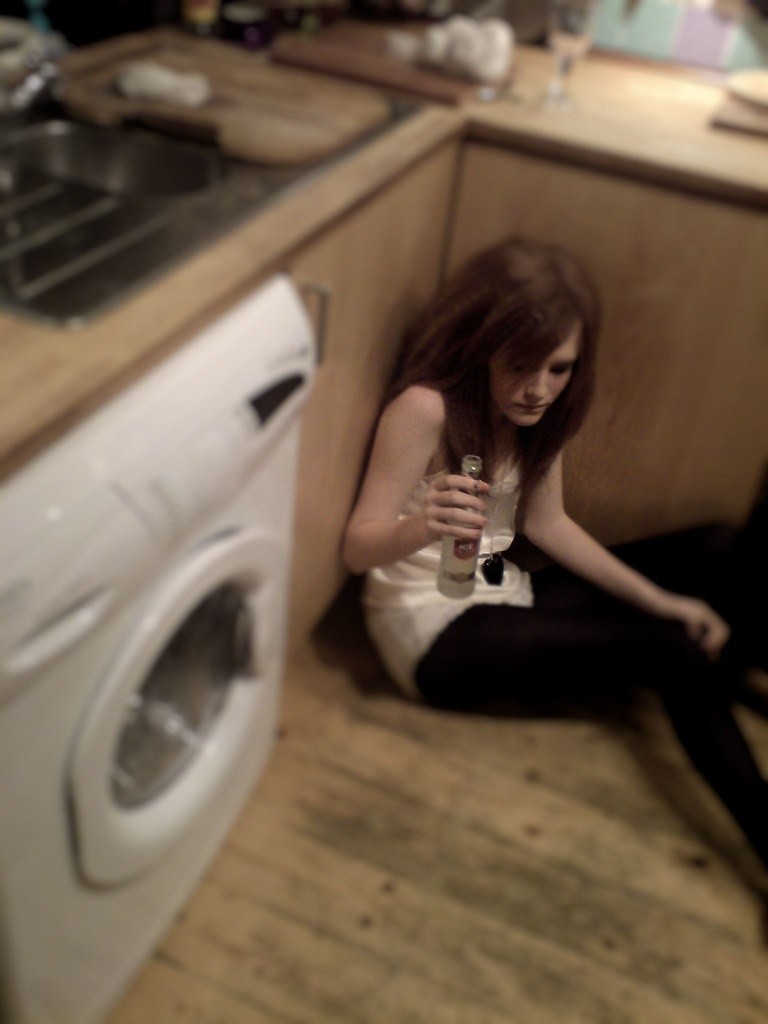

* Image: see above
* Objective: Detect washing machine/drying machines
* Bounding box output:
[0,270,321,1024]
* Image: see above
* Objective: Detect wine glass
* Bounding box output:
[545,0,595,98]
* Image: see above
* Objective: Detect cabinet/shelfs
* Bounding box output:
[57,0,768,671]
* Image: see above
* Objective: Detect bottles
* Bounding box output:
[437,454,484,599]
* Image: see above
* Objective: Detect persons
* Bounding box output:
[340,230,768,874]
